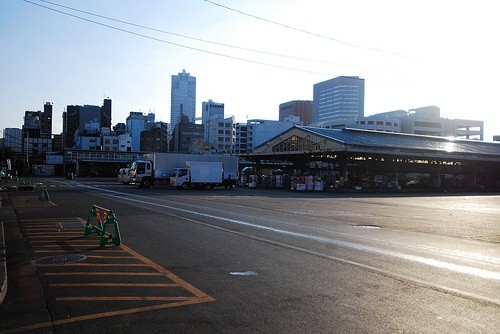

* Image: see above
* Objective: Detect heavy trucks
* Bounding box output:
[129,152,240,191]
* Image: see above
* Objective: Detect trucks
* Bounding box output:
[241,166,273,186]
[169,160,224,190]
[118,168,130,184]
